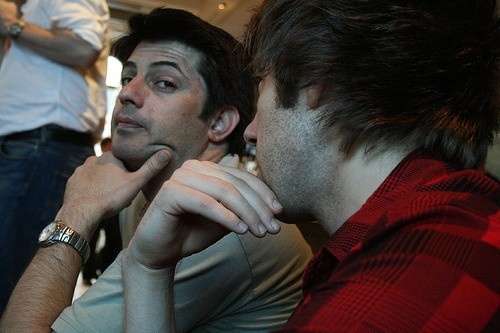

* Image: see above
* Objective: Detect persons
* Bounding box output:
[121,0,500,333]
[1,6,324,333]
[0,0,109,319]
[83,138,121,286]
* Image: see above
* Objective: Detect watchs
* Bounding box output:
[11,18,24,40]
[37,221,90,263]
